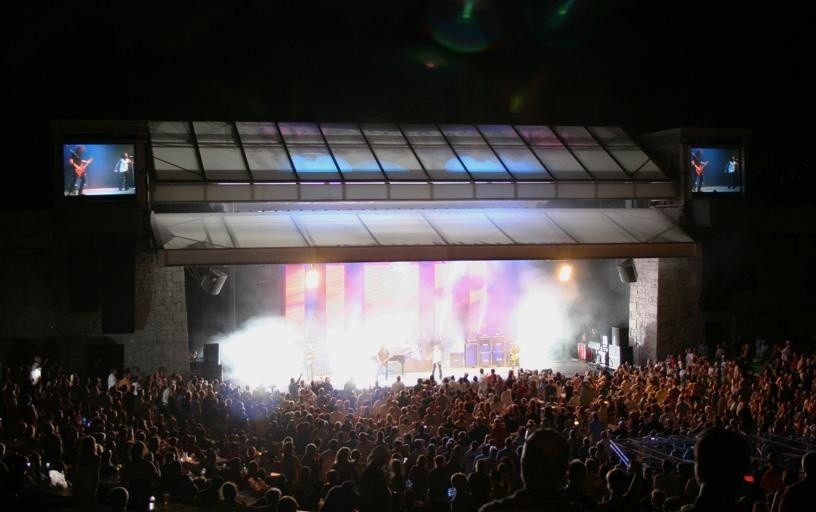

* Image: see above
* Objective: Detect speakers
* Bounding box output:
[611,326,629,346]
[200,269,228,295]
[617,260,639,284]
[608,344,633,369]
[203,343,220,365]
[200,363,223,385]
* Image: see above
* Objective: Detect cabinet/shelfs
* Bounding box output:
[369,354,405,379]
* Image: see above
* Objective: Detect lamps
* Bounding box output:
[305,269,320,289]
[558,263,575,283]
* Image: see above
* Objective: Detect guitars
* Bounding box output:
[696,161,708,175]
[76,159,92,176]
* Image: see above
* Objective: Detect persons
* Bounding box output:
[68,146,93,195]
[0,339,816,511]
[112,152,133,191]
[723,155,739,190]
[691,149,711,192]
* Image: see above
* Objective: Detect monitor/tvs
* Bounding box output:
[54,138,146,207]
[685,140,747,197]
